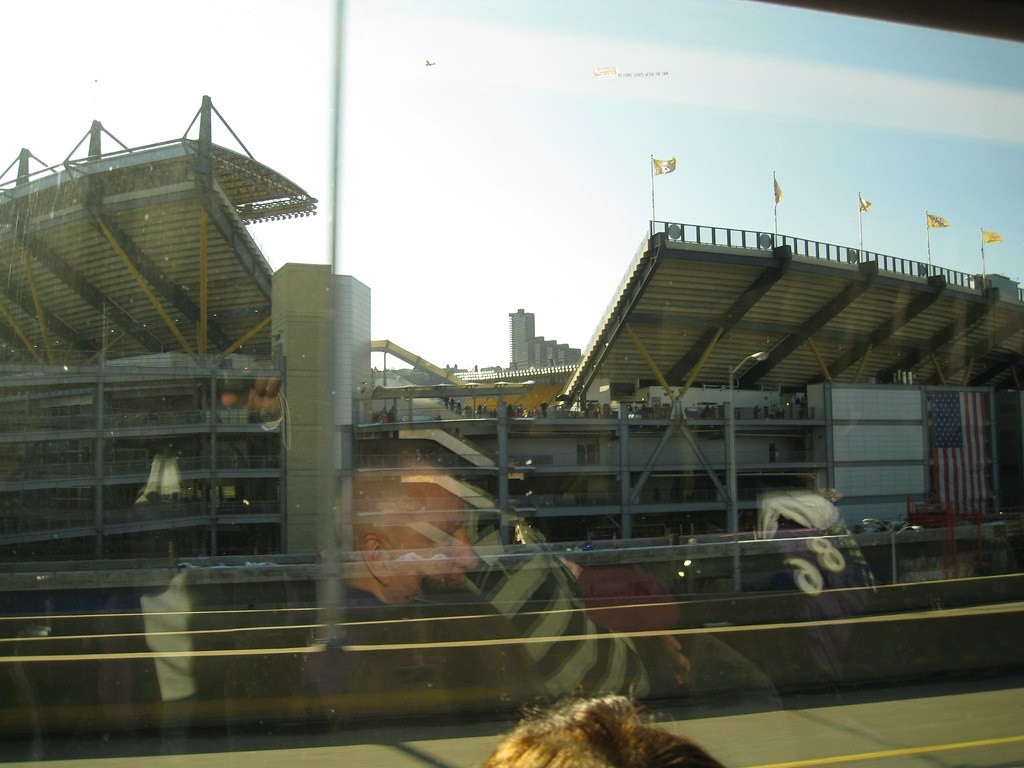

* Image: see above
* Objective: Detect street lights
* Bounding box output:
[862,518,922,586]
[729,350,771,596]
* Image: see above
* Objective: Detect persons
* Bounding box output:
[367,390,812,421]
[128,369,280,753]
[308,431,875,768]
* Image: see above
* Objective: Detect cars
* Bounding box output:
[853,517,914,534]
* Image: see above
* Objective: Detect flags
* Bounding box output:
[860,196,871,215]
[774,178,782,206]
[928,391,993,515]
[980,230,1004,246]
[653,158,677,176]
[928,215,950,232]
